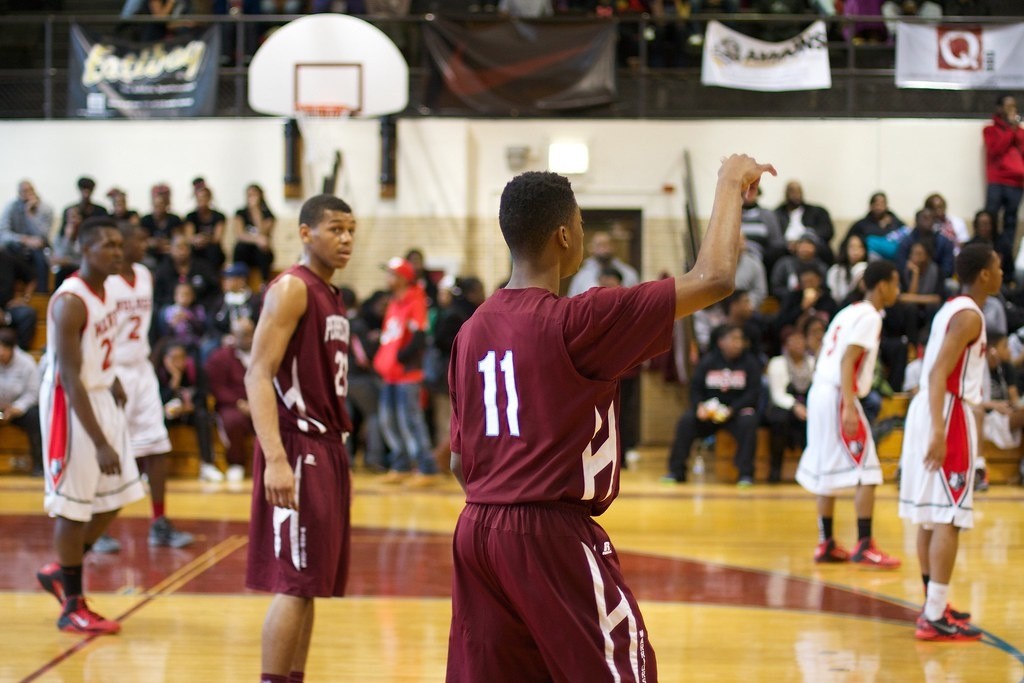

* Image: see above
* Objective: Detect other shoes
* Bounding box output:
[406,471,439,489]
[199,465,222,482]
[380,470,404,484]
[227,464,243,484]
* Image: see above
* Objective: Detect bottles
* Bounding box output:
[1008,114,1020,123]
[164,398,181,419]
[694,456,705,486]
[703,396,729,423]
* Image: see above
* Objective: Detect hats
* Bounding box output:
[379,257,415,282]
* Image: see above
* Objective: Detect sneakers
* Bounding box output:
[56,597,121,635]
[93,536,120,553]
[917,602,971,625]
[147,518,194,547]
[915,602,982,644]
[814,539,850,564]
[37,562,67,606]
[851,538,901,570]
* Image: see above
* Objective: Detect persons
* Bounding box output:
[983,93,1024,243]
[445,151,777,683]
[338,248,485,486]
[36,216,146,634]
[494,0,837,51]
[667,179,1024,490]
[566,226,638,472]
[245,194,357,683]
[882,0,943,44]
[0,174,140,477]
[899,244,1003,642]
[93,222,194,554]
[140,177,279,484]
[794,260,901,571]
